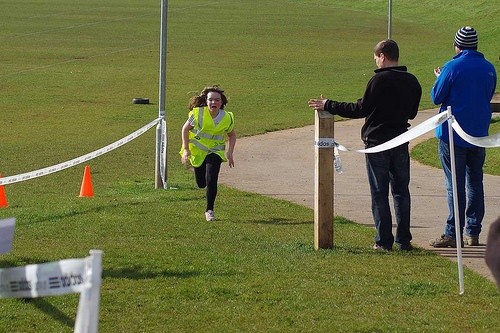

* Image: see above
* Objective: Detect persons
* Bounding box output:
[429,26,497,248]
[308,40,422,253]
[179,85,237,222]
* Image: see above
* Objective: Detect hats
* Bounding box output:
[454,25,478,51]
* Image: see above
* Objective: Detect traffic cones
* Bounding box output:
[0,173,9,208]
[76,165,94,199]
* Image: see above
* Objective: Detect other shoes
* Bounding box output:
[205,209,216,222]
[428,234,464,249]
[396,244,412,253]
[463,234,479,246]
[374,244,392,252]
